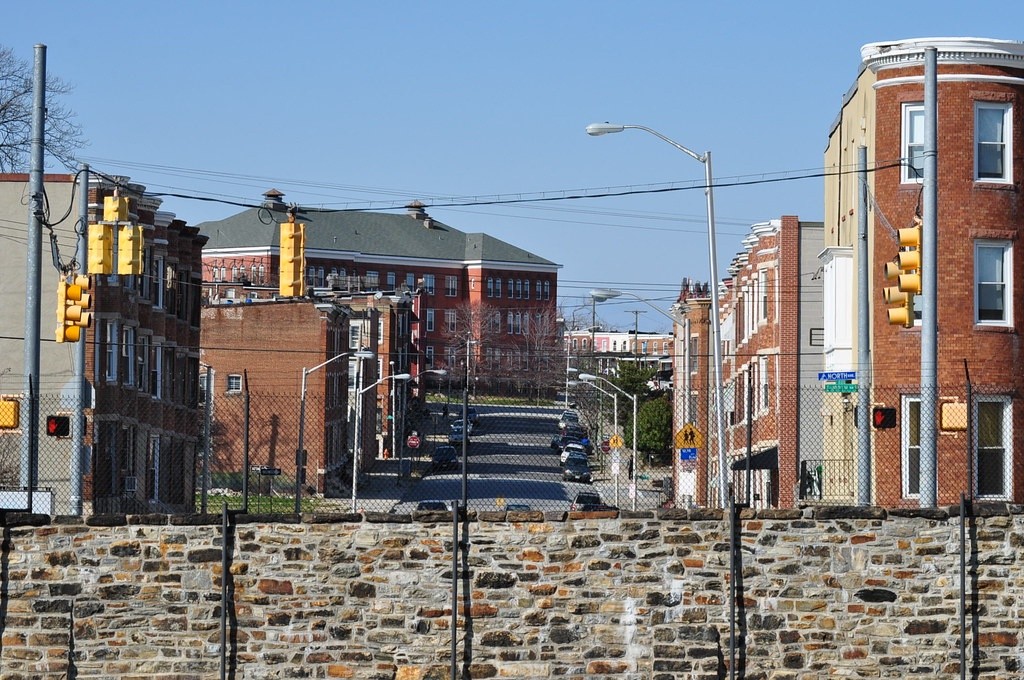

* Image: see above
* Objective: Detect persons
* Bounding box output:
[442,403,449,419]
[626,454,633,479]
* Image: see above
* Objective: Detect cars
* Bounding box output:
[430,445,458,471]
[504,504,529,511]
[414,501,453,511]
[552,409,589,482]
[451,406,480,435]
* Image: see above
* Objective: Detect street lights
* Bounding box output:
[353,373,410,509]
[588,289,692,421]
[567,368,603,473]
[398,370,448,484]
[586,121,729,509]
[579,374,636,511]
[558,318,602,408]
[467,340,477,393]
[567,380,619,508]
[295,351,375,508]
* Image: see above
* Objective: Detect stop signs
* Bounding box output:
[406,436,420,448]
[600,440,611,452]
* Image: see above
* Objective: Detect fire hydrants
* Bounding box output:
[383,449,389,460]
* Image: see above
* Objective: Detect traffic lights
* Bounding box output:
[71,273,92,330]
[56,281,81,342]
[44,415,70,438]
[872,408,897,429]
[884,260,913,329]
[895,225,922,296]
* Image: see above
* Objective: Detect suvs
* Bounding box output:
[569,491,601,511]
